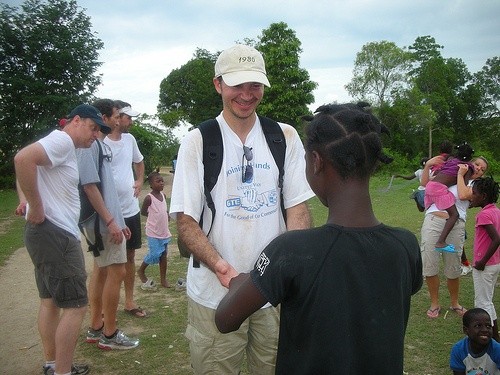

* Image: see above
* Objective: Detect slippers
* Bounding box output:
[100,313,118,323]
[426,306,440,319]
[434,244,459,253]
[450,305,467,316]
[124,306,147,317]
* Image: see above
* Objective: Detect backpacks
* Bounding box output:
[177,114,287,269]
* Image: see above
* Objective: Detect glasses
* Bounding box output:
[242,143,254,183]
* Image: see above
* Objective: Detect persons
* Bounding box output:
[216,102,424,375]
[391,140,500,342]
[449,308,500,375]
[14,103,112,375]
[75,99,174,350]
[169,44,316,375]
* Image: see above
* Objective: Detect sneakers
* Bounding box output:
[96,329,140,351]
[175,278,187,291]
[86,321,105,343]
[43,363,91,375]
[141,279,157,291]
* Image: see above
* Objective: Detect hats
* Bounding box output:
[118,106,141,117]
[69,104,112,134]
[214,45,271,88]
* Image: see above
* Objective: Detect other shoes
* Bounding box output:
[460,265,472,276]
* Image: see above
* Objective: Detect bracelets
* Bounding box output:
[106,218,114,226]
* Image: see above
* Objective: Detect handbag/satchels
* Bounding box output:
[408,183,428,212]
[77,138,105,223]
